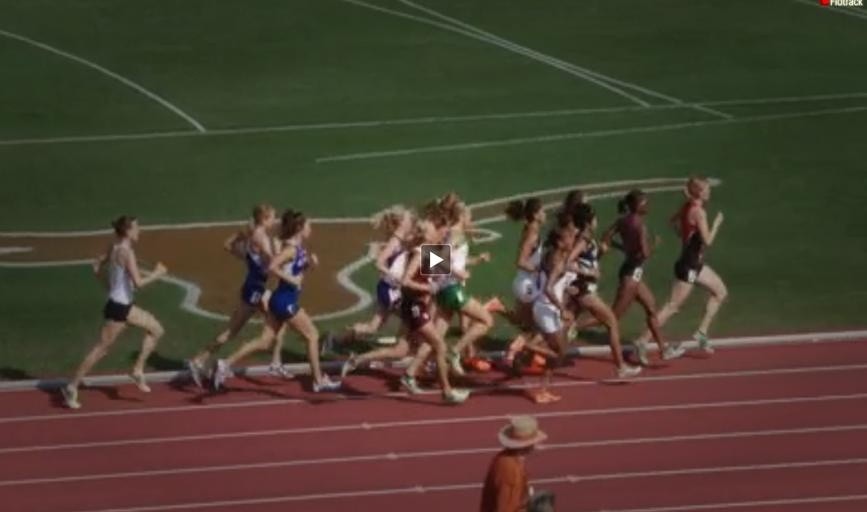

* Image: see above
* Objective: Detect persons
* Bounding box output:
[59,214,169,411]
[188,174,728,402]
[478,409,555,512]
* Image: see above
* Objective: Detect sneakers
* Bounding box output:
[187,355,206,389]
[313,373,342,393]
[127,368,151,392]
[59,382,82,409]
[268,363,296,380]
[634,336,650,366]
[660,345,686,360]
[391,321,580,404]
[693,329,714,354]
[319,330,332,361]
[339,351,358,380]
[213,358,232,392]
[618,366,642,379]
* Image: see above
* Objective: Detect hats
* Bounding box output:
[497,415,548,450]
